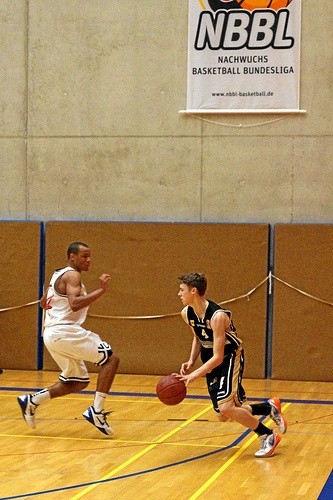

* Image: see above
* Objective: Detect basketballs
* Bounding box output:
[156,374,187,407]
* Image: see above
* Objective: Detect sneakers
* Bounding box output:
[82,405,114,436]
[254,431,281,457]
[17,394,39,429]
[267,396,287,434]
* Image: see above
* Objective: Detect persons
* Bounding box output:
[16,242,119,437]
[171,272,287,458]
